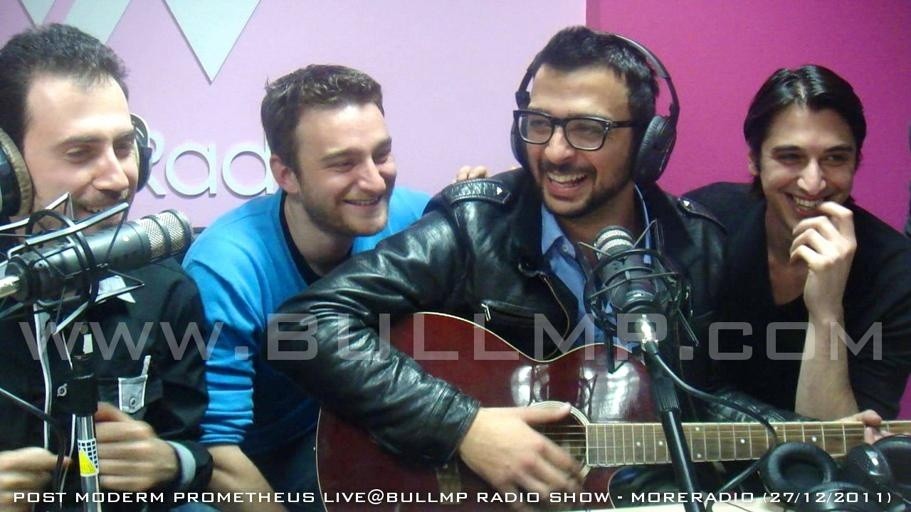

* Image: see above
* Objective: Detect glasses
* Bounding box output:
[513,109,640,151]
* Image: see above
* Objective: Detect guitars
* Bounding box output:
[315,309,911,512]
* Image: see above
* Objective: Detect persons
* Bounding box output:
[181,65,497,512]
[682,64,911,421]
[259,26,894,512]
[0,24,212,512]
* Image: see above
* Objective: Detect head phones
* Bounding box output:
[0,113,153,216]
[510,37,681,188]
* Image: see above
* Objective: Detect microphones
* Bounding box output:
[577,219,685,375]
[0,191,196,317]
